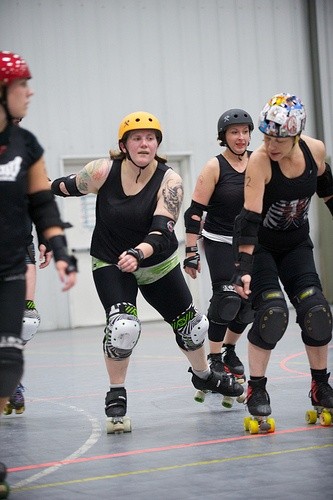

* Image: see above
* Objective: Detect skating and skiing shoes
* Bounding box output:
[221,346,247,382]
[206,355,230,395]
[104,387,132,435]
[186,366,245,408]
[243,377,276,435]
[4,382,26,415]
[305,372,333,426]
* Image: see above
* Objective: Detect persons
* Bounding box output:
[48,111,245,433]
[3,233,40,415]
[184,109,254,403]
[0,51,79,500]
[228,93,333,433]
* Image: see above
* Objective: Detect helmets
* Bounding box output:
[0,49,32,85]
[118,111,163,155]
[258,93,307,137]
[217,108,254,141]
